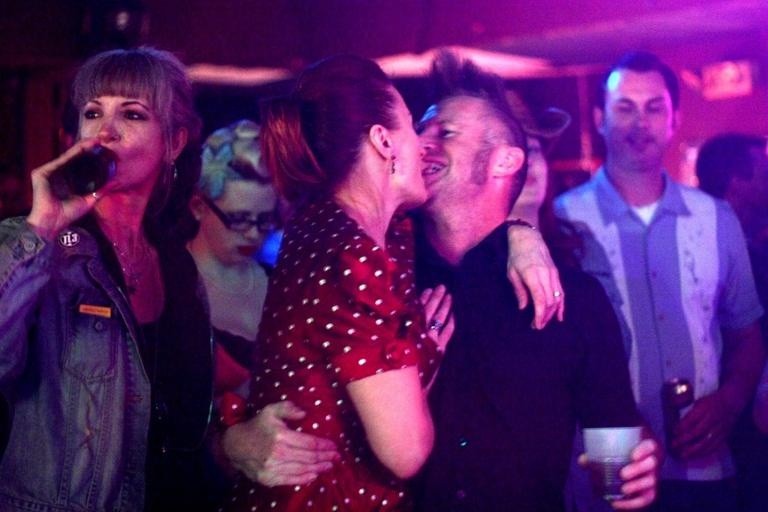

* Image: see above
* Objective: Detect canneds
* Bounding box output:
[662,377,693,458]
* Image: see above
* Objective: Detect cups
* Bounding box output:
[583,426,644,502]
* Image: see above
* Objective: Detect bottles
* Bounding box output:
[62,142,117,198]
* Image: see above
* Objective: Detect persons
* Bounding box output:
[1,47,279,511]
[212,49,662,510]
[552,51,768,510]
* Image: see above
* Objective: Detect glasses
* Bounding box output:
[196,190,285,235]
